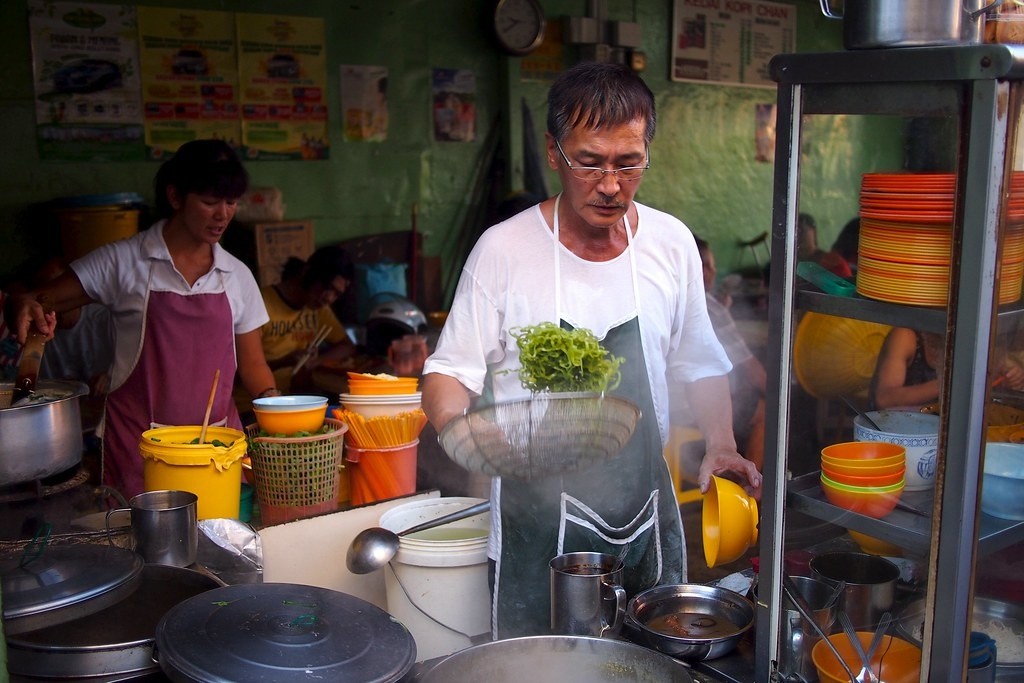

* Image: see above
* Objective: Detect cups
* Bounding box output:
[106,490,198,568]
[843,0,1003,47]
[550,552,626,639]
[387,335,428,374]
[752,554,901,675]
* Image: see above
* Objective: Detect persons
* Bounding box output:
[763,211,861,291]
[421,60,764,645]
[864,324,1023,411]
[0,245,117,460]
[257,243,357,396]
[678,233,768,484]
[2,139,282,514]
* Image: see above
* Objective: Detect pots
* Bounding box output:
[424,635,692,683]
[0,378,91,487]
[6,565,230,683]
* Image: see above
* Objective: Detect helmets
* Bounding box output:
[360,299,428,362]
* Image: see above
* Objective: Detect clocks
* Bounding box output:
[491,0,547,54]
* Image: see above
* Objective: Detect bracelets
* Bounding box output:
[257,387,281,398]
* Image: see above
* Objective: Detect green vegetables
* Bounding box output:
[150,437,236,448]
[248,424,339,508]
[495,321,625,393]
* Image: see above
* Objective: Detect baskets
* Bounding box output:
[245,416,349,528]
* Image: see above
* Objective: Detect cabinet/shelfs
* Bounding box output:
[752,34,1024,683]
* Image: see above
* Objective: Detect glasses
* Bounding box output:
[555,136,653,180]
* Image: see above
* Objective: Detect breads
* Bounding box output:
[985,0,1024,44]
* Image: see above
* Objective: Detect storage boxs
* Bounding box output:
[229,187,314,288]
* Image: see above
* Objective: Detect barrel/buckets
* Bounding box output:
[139,426,247,518]
[379,496,494,662]
[343,438,419,506]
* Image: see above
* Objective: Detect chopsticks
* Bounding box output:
[331,407,427,446]
[291,324,332,375]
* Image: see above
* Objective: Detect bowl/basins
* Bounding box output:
[892,597,1024,683]
[813,632,922,683]
[701,474,760,568]
[252,395,328,433]
[628,584,757,659]
[339,369,426,417]
[819,404,1023,525]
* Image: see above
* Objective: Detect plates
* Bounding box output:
[856,173,1023,310]
